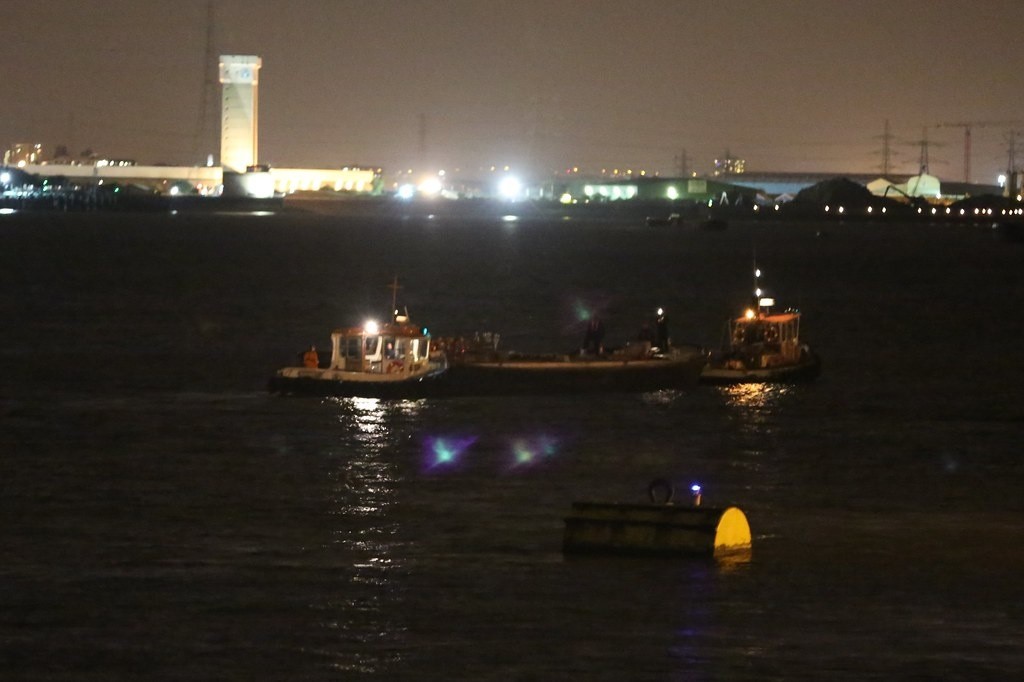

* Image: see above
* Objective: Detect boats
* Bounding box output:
[447,304,707,403]
[559,477,730,559]
[699,215,728,232]
[697,263,823,386]
[646,213,685,226]
[274,272,450,402]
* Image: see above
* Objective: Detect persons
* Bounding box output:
[303,345,319,368]
[656,307,674,354]
[582,308,603,355]
[385,342,394,358]
